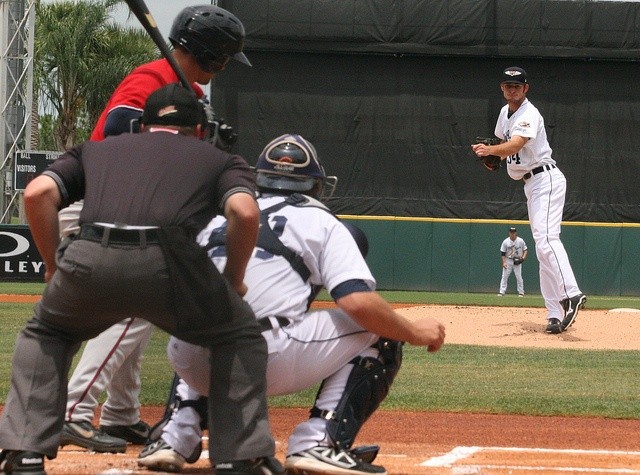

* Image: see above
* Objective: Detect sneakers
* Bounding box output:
[545,318,562,333]
[497,293,503,296]
[137,437,186,472]
[559,294,586,331]
[215,456,285,475]
[0,449,45,474]
[519,294,524,297]
[100,420,153,445]
[285,445,386,475]
[59,421,127,454]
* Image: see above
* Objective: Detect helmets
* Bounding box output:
[254,133,337,204]
[169,4,252,72]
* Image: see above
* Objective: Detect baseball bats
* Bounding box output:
[124,0,232,151]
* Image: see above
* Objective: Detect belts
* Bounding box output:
[256,315,288,330]
[77,224,162,243]
[523,164,556,180]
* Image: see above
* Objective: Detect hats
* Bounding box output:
[142,83,205,126]
[501,66,528,85]
[509,227,517,232]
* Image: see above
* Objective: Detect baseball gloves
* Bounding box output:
[474,137,503,171]
[514,257,523,265]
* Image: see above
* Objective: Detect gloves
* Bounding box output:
[198,95,219,121]
[209,118,237,153]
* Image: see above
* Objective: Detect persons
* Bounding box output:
[471,67,587,333]
[498,228,528,297]
[138,132,446,474]
[61,5,252,456]
[0,82,282,474]
